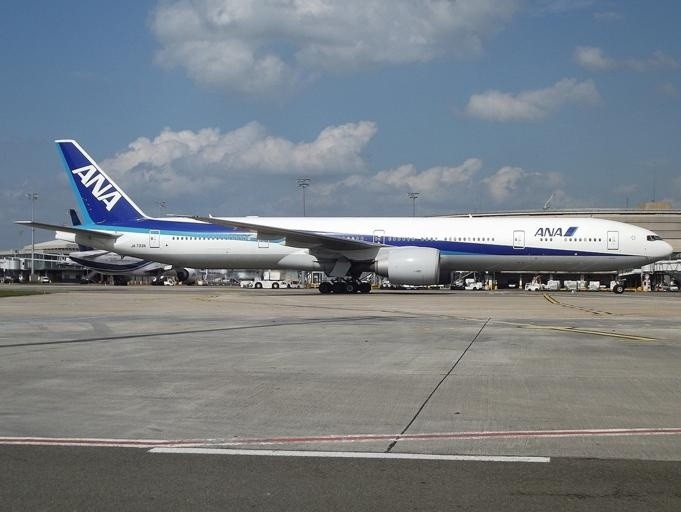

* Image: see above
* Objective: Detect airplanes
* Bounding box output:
[14,139,673,295]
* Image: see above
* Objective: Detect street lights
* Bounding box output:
[23,193,40,280]
[407,191,421,217]
[295,177,311,216]
